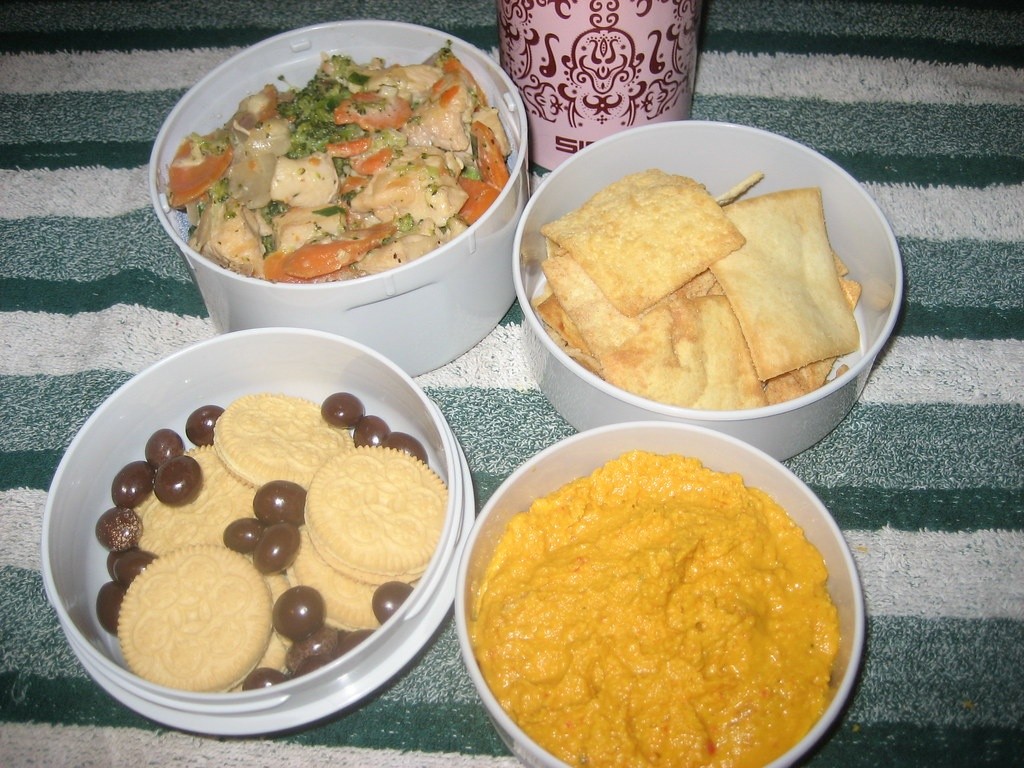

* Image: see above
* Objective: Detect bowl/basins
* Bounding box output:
[146,19,533,381]
[450,418,867,767]
[514,117,905,463]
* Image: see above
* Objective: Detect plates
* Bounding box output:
[42,328,477,735]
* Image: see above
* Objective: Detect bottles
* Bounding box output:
[497,0,703,172]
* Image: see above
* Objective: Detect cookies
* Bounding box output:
[117,392,447,694]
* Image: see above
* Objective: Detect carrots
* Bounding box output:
[167,59,508,286]
